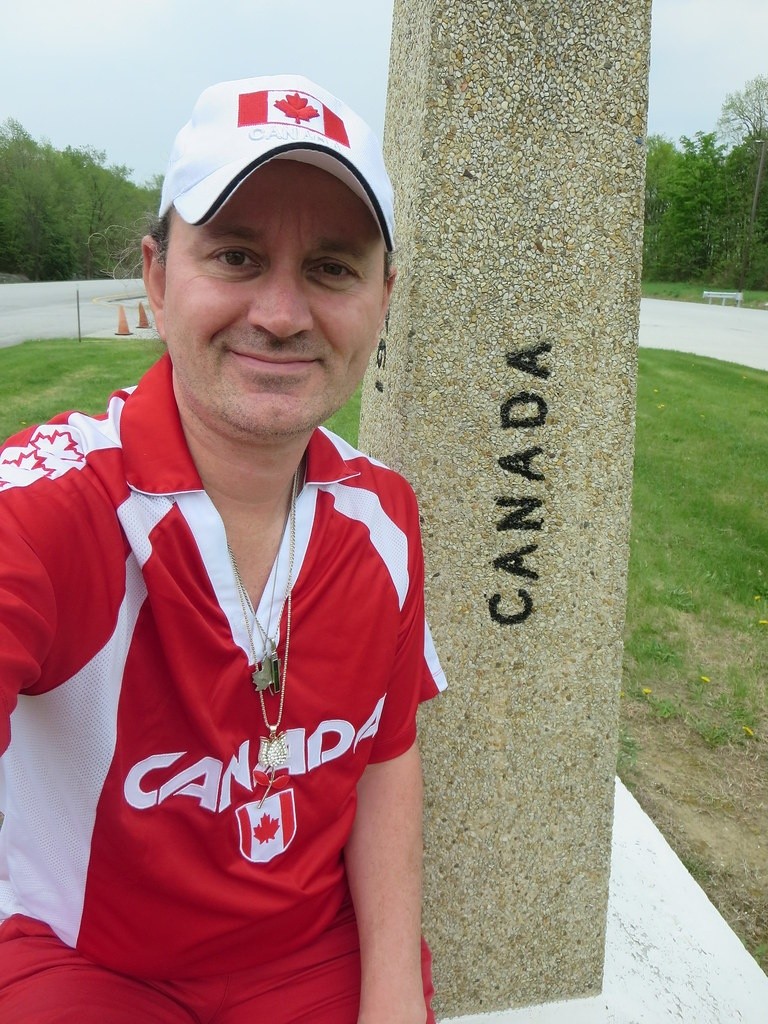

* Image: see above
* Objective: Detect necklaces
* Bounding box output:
[227,467,301,807]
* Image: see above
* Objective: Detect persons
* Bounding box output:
[0,73,448,1024]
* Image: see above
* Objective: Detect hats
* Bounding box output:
[158,76,395,252]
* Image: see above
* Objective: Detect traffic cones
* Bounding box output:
[115,304,133,335]
[135,302,154,329]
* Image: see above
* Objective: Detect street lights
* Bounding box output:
[750,138,766,221]
